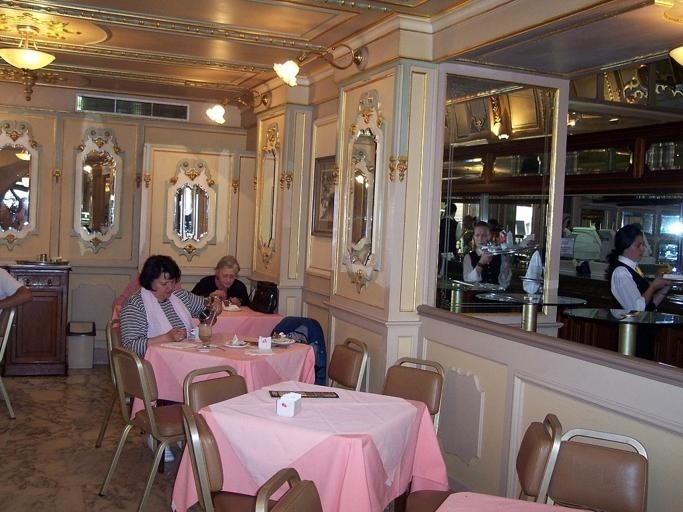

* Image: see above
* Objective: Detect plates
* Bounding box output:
[271,337,295,344]
[161,340,196,349]
[223,304,239,312]
[223,340,249,348]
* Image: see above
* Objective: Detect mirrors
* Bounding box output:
[257,124,280,268]
[164,160,218,262]
[71,127,123,253]
[344,91,383,293]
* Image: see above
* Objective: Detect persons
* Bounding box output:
[609,224,671,312]
[523,215,547,293]
[0,265,33,346]
[440,205,511,289]
[191,255,250,307]
[120,255,222,462]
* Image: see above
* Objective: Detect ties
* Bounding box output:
[636,266,644,278]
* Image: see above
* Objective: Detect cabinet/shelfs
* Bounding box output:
[0,262,68,376]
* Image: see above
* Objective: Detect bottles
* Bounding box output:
[499,229,513,247]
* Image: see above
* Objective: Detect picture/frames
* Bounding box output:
[311,155,337,237]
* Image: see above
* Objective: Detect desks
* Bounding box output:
[435,490,576,511]
[208,305,284,335]
[172,378,449,510]
[437,282,683,354]
[131,333,317,421]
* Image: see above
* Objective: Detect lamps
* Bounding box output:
[206,91,271,123]
[0,32,55,70]
[103,178,112,193]
[16,150,31,160]
[273,41,369,87]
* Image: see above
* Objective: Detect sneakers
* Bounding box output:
[147,434,175,462]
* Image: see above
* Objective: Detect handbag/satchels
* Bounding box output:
[245,281,279,314]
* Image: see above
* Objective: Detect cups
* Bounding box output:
[40,253,47,262]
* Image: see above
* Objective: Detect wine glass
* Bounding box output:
[195,323,213,350]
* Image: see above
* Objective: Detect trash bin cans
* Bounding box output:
[66,321,96,369]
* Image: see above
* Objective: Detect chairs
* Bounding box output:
[100,349,186,512]
[406,413,562,511]
[272,317,325,385]
[97,319,126,446]
[382,357,447,500]
[548,428,646,509]
[183,365,248,431]
[111,282,140,324]
[326,337,371,395]
[256,467,324,512]
[183,403,277,510]
[0,307,17,419]
